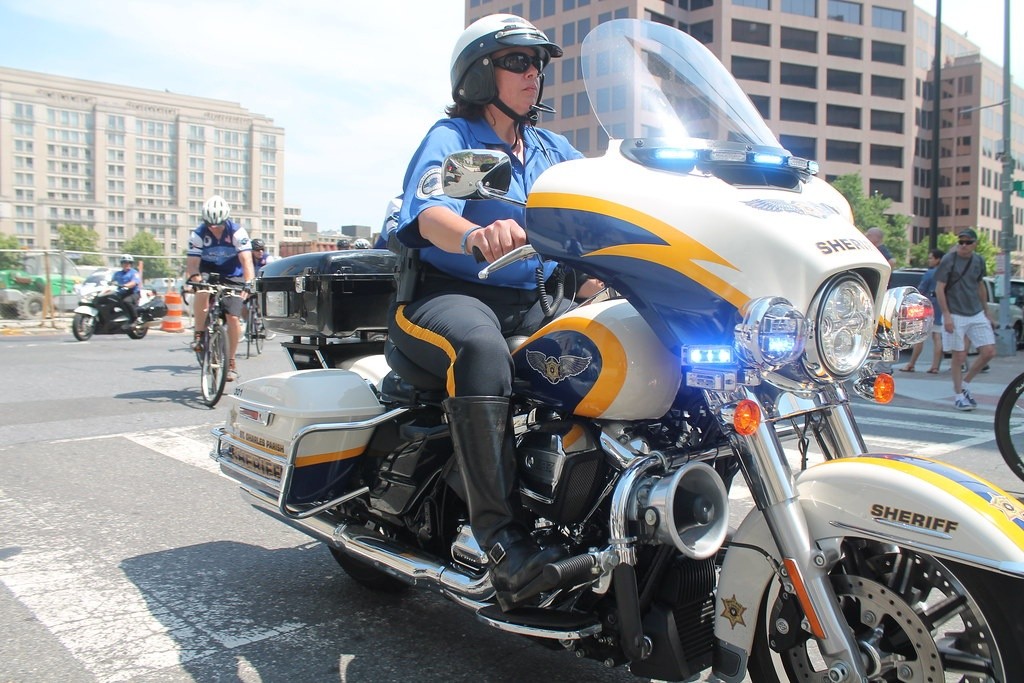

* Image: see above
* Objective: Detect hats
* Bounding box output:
[959,229,978,239]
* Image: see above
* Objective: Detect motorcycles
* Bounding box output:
[72,267,168,342]
[208,14,1024,683]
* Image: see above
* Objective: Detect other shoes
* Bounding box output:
[952,399,974,411]
[962,389,977,409]
[225,369,239,382]
[981,365,991,373]
[264,330,276,340]
[237,320,248,344]
[191,334,205,353]
[947,363,967,372]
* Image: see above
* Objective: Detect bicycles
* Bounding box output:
[242,291,267,360]
[179,271,259,408]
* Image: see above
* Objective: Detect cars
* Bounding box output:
[885,266,1024,351]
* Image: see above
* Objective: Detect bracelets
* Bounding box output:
[461,226,481,257]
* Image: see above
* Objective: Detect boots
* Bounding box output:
[125,302,138,325]
[442,395,571,613]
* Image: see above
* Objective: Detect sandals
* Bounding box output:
[926,367,939,373]
[899,365,914,372]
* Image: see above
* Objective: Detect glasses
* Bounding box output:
[493,53,544,74]
[958,239,976,246]
[252,247,265,252]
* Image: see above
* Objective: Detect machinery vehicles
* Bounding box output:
[0,252,83,321]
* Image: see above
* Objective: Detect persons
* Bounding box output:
[111,253,141,331]
[186,195,254,382]
[899,249,946,374]
[374,193,405,249]
[337,238,350,250]
[239,238,276,342]
[933,229,997,410]
[355,238,370,249]
[949,336,991,373]
[390,13,607,612]
[865,227,897,266]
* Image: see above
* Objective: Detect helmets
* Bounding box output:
[202,195,230,225]
[252,239,265,249]
[120,254,134,262]
[450,12,564,102]
